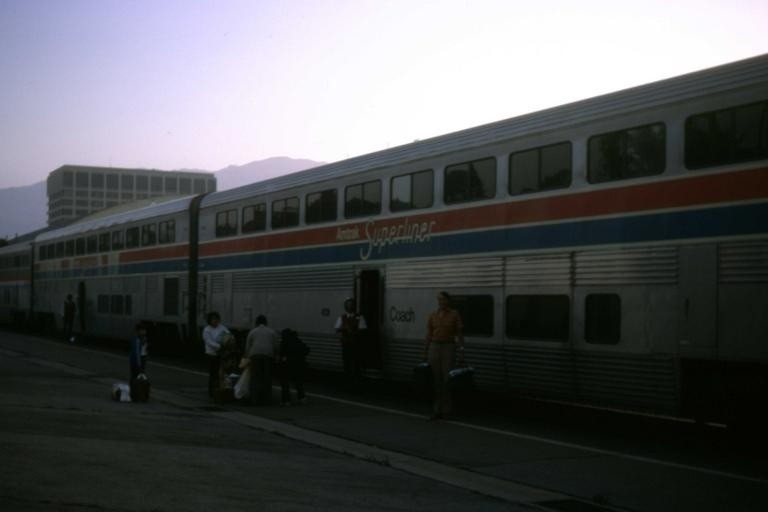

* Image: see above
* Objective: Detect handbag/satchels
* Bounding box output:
[218,333,236,360]
[112,383,133,403]
[131,372,150,402]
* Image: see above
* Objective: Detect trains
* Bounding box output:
[0,50,768,455]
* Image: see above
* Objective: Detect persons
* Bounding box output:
[128,323,150,380]
[233,358,252,399]
[234,315,277,401]
[333,297,368,388]
[423,290,467,421]
[201,310,236,397]
[62,293,77,336]
[271,324,312,408]
